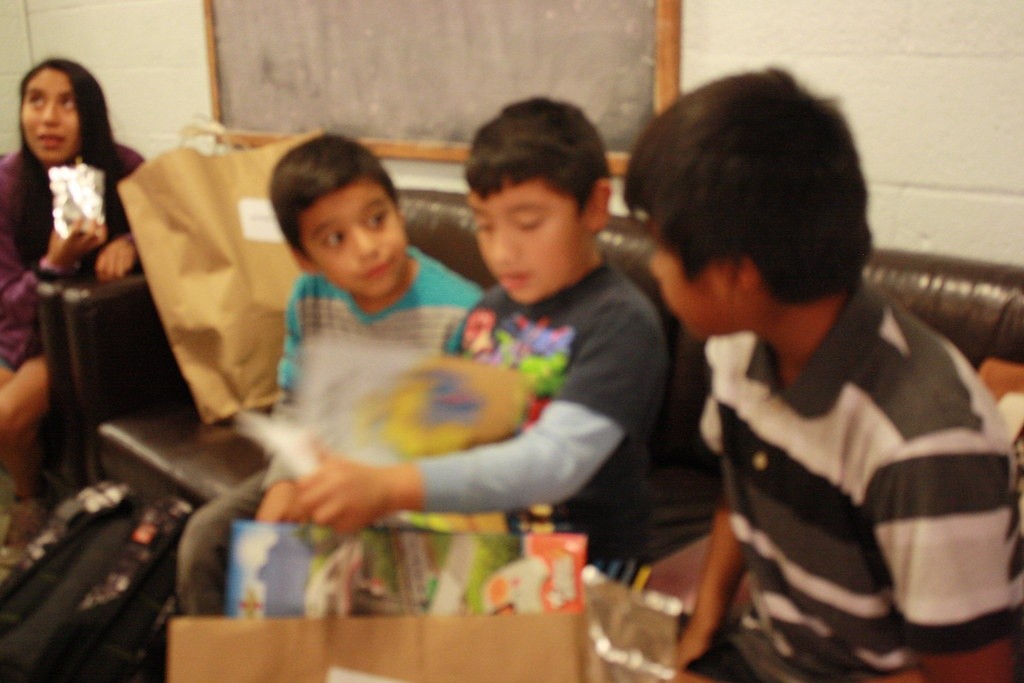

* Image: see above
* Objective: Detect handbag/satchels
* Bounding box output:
[117,115,323,424]
[165,518,587,683]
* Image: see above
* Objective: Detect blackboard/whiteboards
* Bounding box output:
[202,0,683,176]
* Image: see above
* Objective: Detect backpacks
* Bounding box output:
[0,480,193,683]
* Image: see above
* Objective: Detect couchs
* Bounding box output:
[34,188,1024,516]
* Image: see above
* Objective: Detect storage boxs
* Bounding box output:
[160,610,592,682]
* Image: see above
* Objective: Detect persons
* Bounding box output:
[0,58,145,554]
[290,96,726,635]
[179,133,482,619]
[625,67,1023,683]
